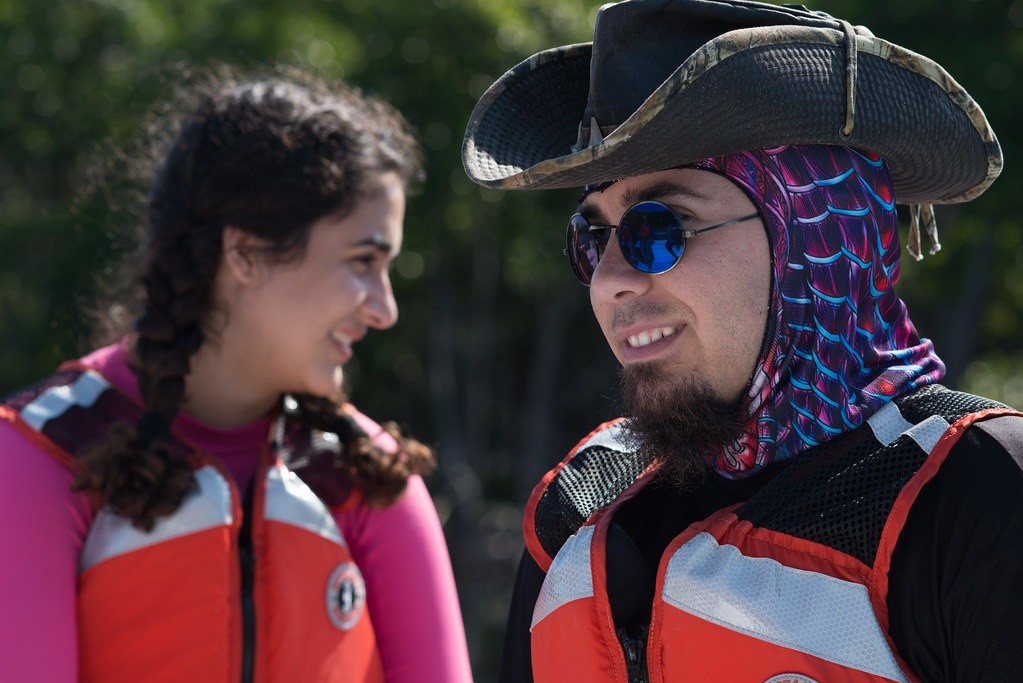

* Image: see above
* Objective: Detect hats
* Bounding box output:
[462,0,1003,264]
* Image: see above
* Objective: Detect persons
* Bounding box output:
[0,65,478,683]
[458,1,1023,683]
[620,215,656,264]
[665,217,684,263]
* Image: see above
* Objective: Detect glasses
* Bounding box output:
[562,201,762,286]
